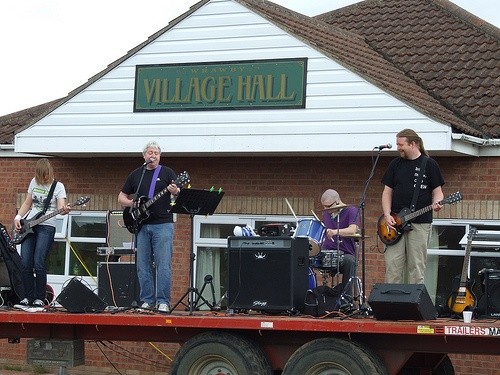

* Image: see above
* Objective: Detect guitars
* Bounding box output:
[446,227,479,313]
[11,196,91,244]
[377,191,463,245]
[122,170,190,235]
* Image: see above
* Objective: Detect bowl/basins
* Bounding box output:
[123,242,133,248]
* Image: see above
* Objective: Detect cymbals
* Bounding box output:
[314,203,360,212]
[341,234,372,240]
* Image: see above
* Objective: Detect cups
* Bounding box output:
[462,311,473,323]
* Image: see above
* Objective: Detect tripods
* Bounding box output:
[339,152,373,320]
[167,189,217,315]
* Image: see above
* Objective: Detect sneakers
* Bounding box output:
[13,297,32,310]
[137,302,155,313]
[158,303,170,312]
[27,298,47,312]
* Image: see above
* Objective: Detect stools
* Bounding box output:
[326,268,343,288]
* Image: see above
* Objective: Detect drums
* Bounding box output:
[293,218,326,256]
[308,266,317,289]
[310,250,344,268]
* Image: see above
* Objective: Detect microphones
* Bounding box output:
[375,144,392,149]
[484,268,500,273]
[142,158,154,168]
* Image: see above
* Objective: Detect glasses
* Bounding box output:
[325,201,336,208]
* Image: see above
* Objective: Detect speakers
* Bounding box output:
[56,277,107,313]
[106,210,134,247]
[226,236,309,313]
[368,283,435,320]
[98,262,158,310]
[436,254,500,319]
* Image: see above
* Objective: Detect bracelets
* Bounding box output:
[14,214,21,220]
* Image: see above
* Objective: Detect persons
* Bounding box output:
[118,142,180,313]
[381,129,446,284]
[320,188,360,310]
[14,158,72,312]
